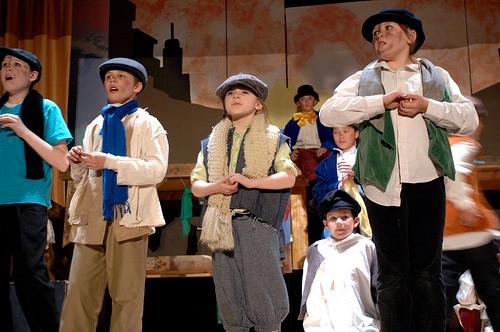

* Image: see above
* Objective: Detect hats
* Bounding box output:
[318,189,362,220]
[293,85,320,106]
[0,45,41,83]
[464,96,487,115]
[353,123,358,129]
[98,58,147,93]
[362,8,425,54]
[216,74,269,102]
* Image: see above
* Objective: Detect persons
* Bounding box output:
[0,45,73,332]
[319,9,479,332]
[313,125,372,239]
[453,270,494,332]
[190,73,298,332]
[441,97,500,332]
[283,85,338,188]
[297,190,382,332]
[59,58,169,332]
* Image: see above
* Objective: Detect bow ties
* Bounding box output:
[293,111,318,126]
